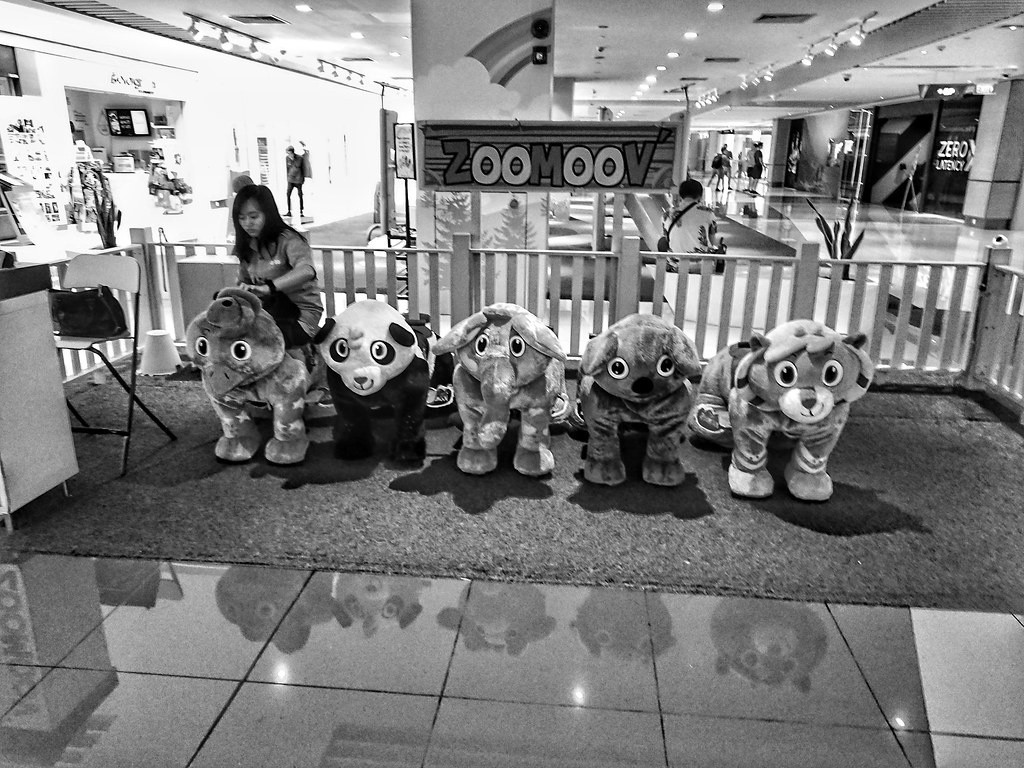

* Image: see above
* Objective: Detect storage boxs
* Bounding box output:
[154,128,176,133]
[153,115,167,125]
[110,154,136,173]
[92,147,108,163]
[165,101,182,126]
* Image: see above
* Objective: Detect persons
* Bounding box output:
[743,142,767,194]
[282,145,305,218]
[707,144,733,192]
[735,151,746,177]
[213,184,323,350]
[662,180,718,275]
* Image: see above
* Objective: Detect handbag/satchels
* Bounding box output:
[714,237,727,273]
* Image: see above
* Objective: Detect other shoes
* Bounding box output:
[750,190,758,195]
[744,189,751,192]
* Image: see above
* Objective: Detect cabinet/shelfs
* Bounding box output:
[0,261,81,535]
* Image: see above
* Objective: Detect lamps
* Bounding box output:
[248,40,263,62]
[700,95,708,106]
[706,94,713,105]
[360,76,365,85]
[185,20,204,43]
[801,47,816,66]
[751,73,763,86]
[849,23,867,47]
[219,31,234,52]
[740,77,750,91]
[346,71,353,81]
[711,91,720,103]
[695,98,702,109]
[317,61,325,73]
[824,37,839,57]
[331,65,338,77]
[763,67,776,82]
[272,49,290,63]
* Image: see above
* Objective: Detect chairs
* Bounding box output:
[53,254,178,478]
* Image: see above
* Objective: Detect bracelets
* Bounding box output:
[265,279,276,296]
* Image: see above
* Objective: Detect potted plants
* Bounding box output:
[806,195,867,280]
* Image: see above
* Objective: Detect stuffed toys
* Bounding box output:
[185,287,325,464]
[569,313,702,486]
[685,319,874,500]
[432,303,568,475]
[312,299,453,463]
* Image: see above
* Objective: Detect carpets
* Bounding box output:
[0,360,1024,618]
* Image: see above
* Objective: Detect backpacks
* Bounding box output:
[711,156,723,169]
